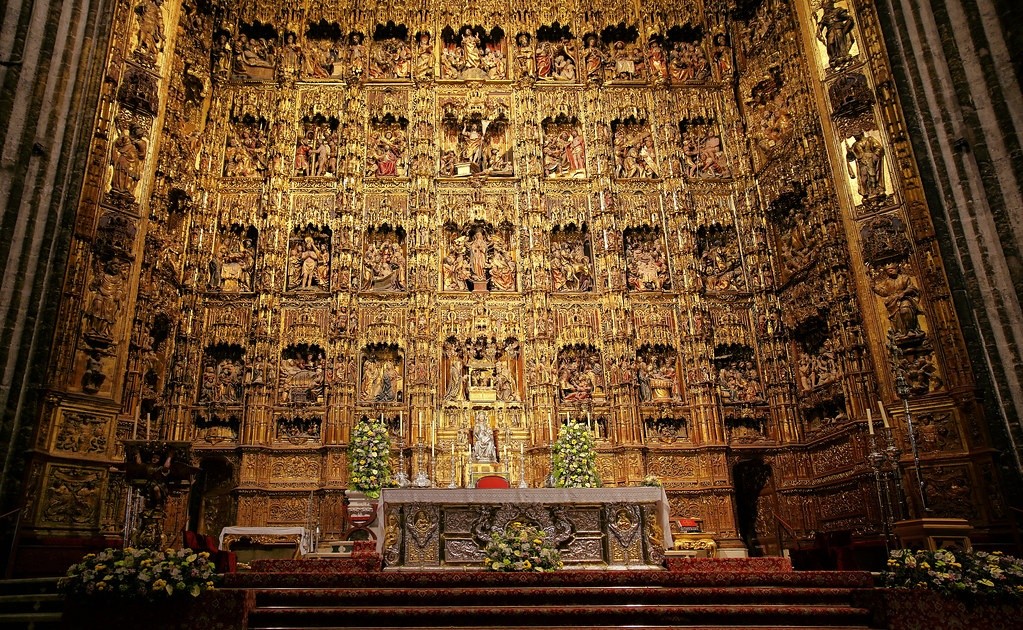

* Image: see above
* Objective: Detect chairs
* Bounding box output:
[790,526,895,571]
[184,530,236,573]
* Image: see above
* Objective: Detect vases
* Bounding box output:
[345,489,376,525]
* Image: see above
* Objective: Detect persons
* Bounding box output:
[110,123,146,199]
[870,262,923,338]
[846,128,882,201]
[135,0,165,61]
[210,27,709,81]
[214,228,738,292]
[473,412,497,460]
[817,0,853,63]
[226,118,722,178]
[86,258,124,336]
[199,344,766,404]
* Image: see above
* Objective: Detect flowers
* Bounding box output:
[641,475,664,487]
[346,419,400,500]
[880,547,1023,598]
[55,548,216,598]
[484,522,564,573]
[552,420,603,488]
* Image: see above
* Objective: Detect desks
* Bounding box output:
[218,525,306,567]
[378,486,673,570]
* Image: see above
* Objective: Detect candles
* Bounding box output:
[532,433,535,445]
[866,408,874,435]
[878,401,889,427]
[381,410,471,458]
[503,443,524,456]
[548,412,591,440]
